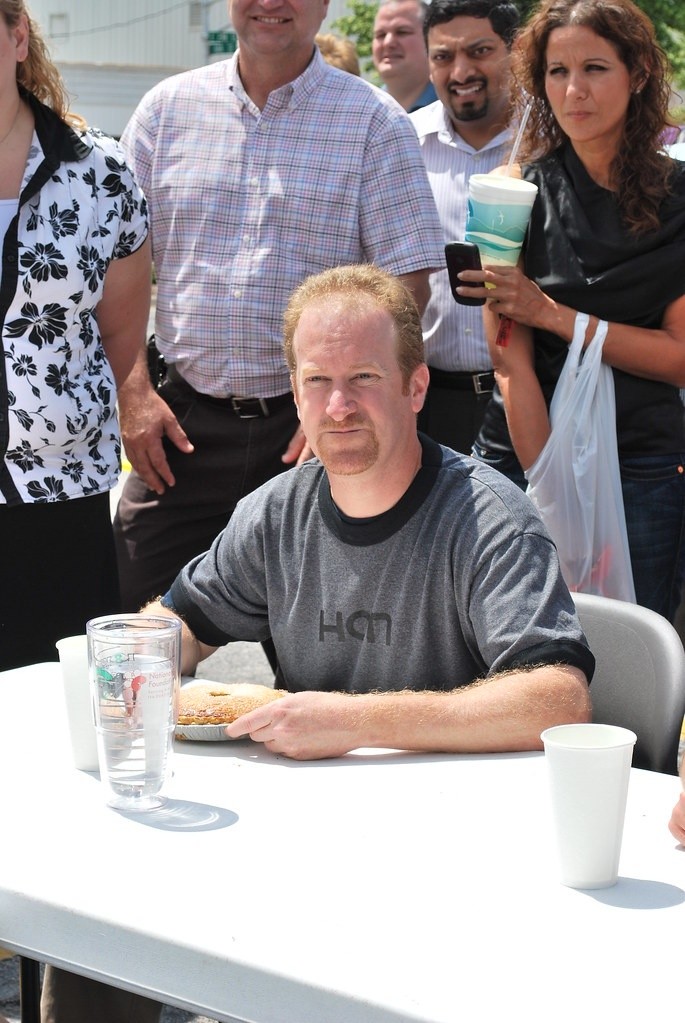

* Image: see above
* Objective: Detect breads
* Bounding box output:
[174,678,291,726]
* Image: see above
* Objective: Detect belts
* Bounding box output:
[428,366,495,395]
[167,365,294,419]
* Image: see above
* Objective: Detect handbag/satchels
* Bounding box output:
[524,312,638,605]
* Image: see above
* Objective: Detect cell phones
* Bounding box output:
[445,242,486,307]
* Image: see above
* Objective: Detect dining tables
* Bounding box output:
[0,662,685,1023]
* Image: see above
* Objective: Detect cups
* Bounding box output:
[56,634,99,772]
[86,614,182,812]
[541,723,637,890]
[464,174,538,290]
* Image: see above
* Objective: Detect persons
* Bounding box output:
[314,34,360,76]
[0,0,153,670]
[370,0,438,114]
[455,0,685,623]
[41,263,598,1023]
[96,0,447,613]
[408,0,561,456]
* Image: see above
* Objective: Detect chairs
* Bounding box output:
[567,591,685,773]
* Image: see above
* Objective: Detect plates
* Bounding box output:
[175,725,247,740]
